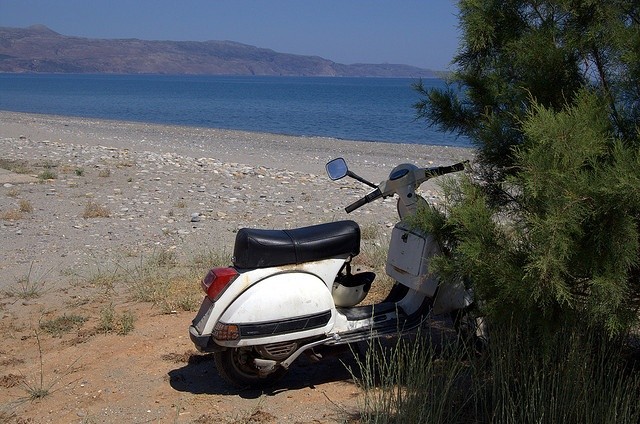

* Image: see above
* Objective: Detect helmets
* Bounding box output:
[332,256,376,308]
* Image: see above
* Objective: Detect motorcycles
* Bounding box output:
[190,157,491,389]
